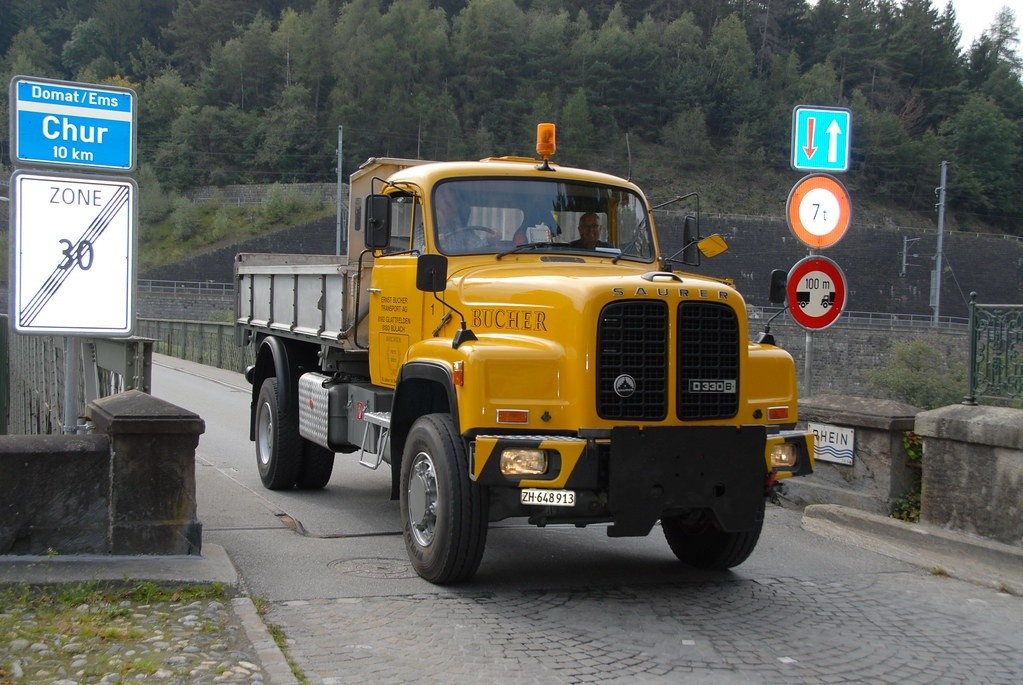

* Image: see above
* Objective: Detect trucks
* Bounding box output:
[231,120,814,588]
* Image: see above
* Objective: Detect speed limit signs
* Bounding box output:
[7,169,138,340]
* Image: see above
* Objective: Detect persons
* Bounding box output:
[569,212,614,250]
[416,188,502,254]
